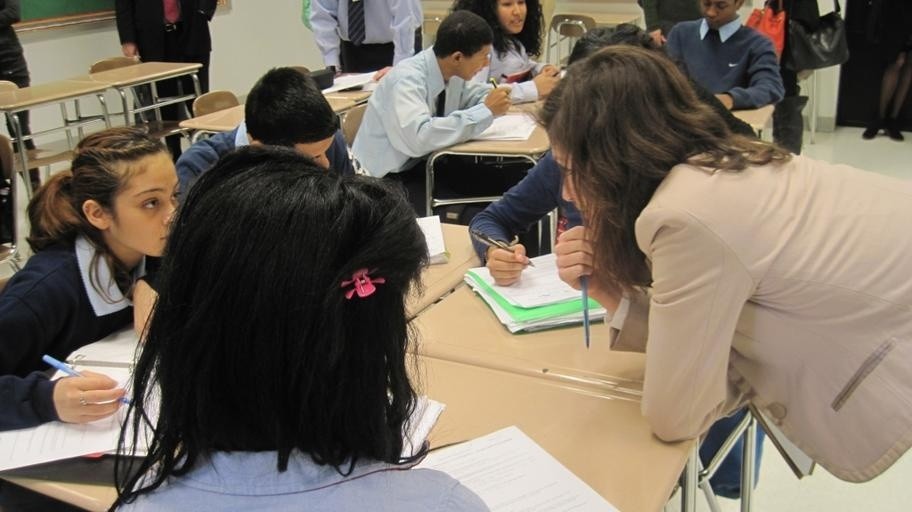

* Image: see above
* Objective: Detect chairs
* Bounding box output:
[0,2,817,260]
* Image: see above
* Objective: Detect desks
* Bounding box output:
[406,282,758,512]
[1,340,167,512]
[402,222,486,320]
[395,353,702,510]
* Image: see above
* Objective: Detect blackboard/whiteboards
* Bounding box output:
[10,0,229,33]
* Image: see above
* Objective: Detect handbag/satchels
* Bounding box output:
[782,0,850,73]
[744,0,786,66]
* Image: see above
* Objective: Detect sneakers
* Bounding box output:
[862,118,905,141]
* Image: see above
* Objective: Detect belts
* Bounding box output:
[158,22,180,32]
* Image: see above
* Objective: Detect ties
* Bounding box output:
[436,89,446,117]
[348,0,366,47]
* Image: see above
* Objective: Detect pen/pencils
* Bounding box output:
[42,354,137,406]
[582,276,590,348]
[490,78,498,88]
[471,230,535,267]
